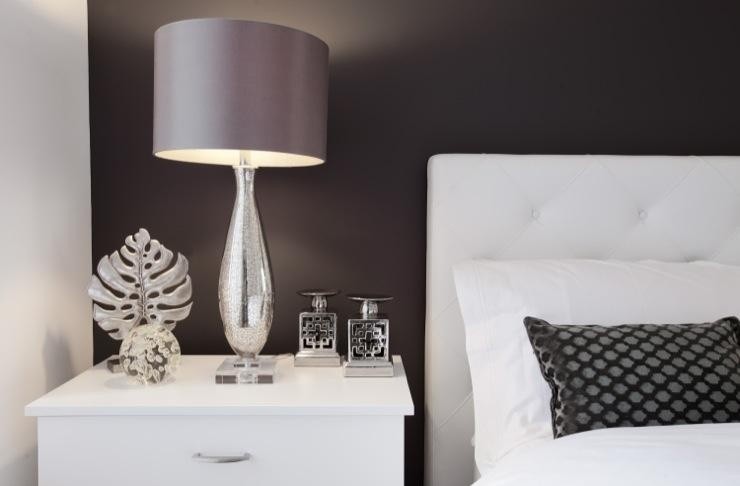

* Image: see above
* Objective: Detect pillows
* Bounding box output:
[519,312,740,438]
[449,259,740,480]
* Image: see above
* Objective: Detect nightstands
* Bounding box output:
[26,351,414,486]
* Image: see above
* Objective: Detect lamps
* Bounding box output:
[149,16,330,384]
